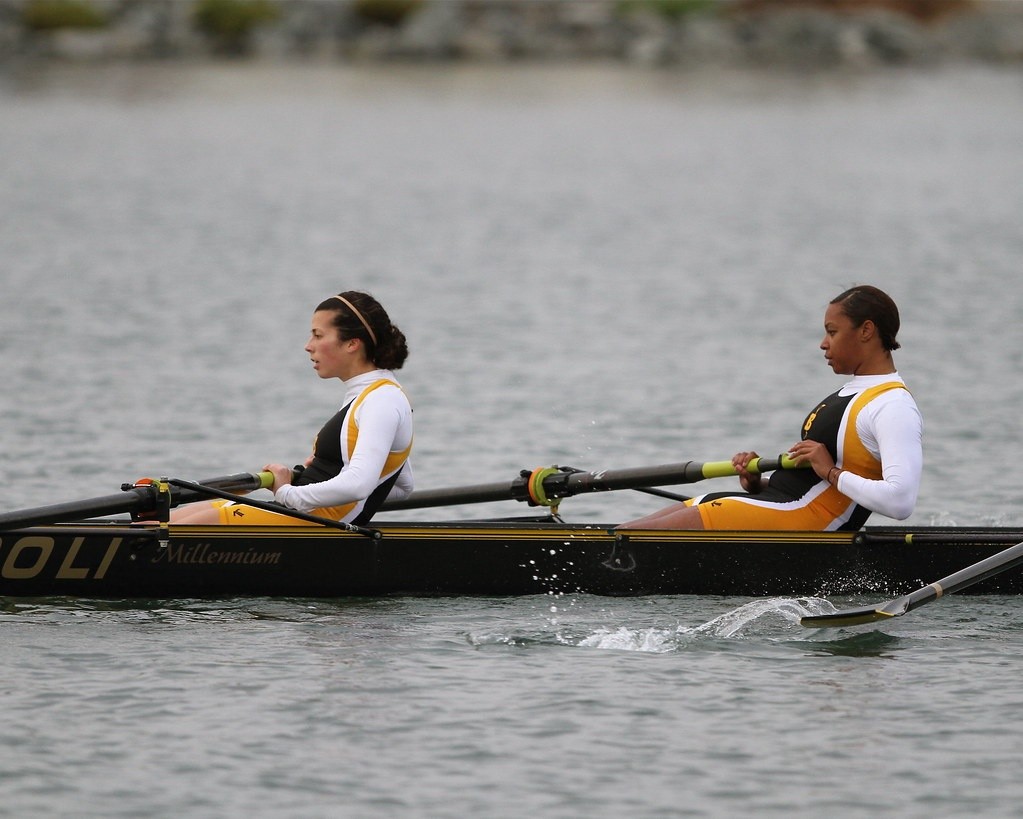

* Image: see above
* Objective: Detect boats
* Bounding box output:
[1,453,1023,628]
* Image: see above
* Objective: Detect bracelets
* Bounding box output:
[827,467,841,485]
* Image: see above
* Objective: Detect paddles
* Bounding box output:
[799,541,1023,629]
[376,452,813,513]
[1,462,307,533]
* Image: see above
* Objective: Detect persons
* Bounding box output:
[132,291,414,526]
[613,285,923,531]
